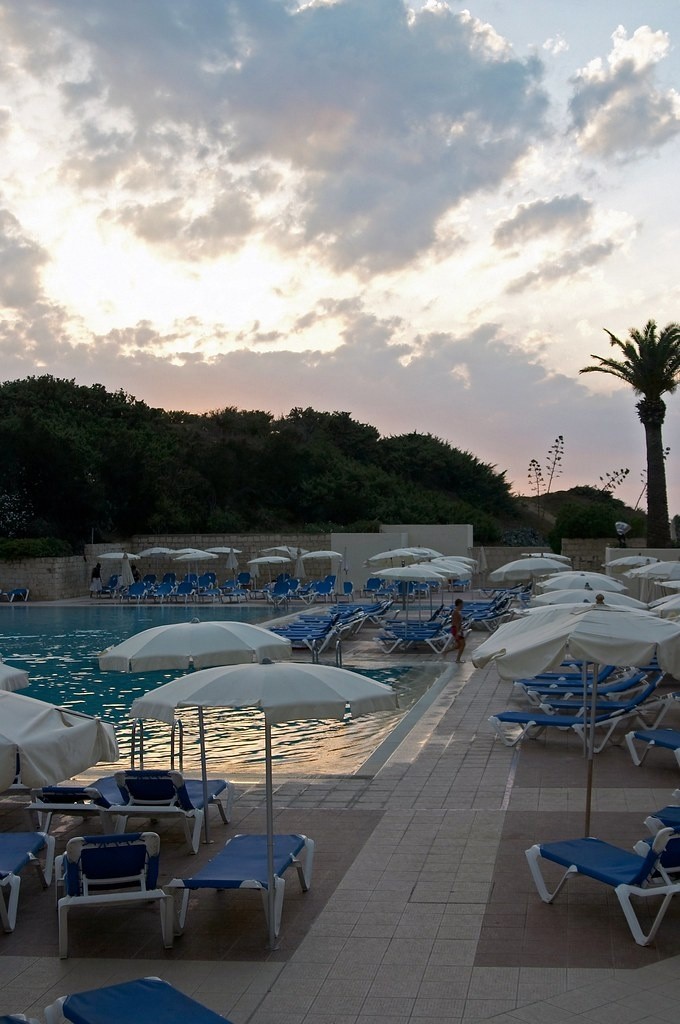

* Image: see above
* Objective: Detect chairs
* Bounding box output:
[0,572,680,959]
[44,976,230,1024]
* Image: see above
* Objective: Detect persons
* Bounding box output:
[89,563,103,599]
[131,565,142,583]
[450,599,467,665]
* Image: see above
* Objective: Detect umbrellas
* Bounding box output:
[0,691,122,796]
[0,663,31,692]
[99,618,293,847]
[471,547,679,842]
[97,545,476,638]
[129,658,388,952]
